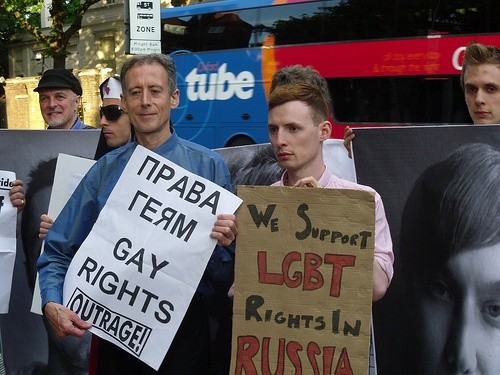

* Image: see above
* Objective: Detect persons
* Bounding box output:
[38,76,174,241]
[33,68,102,130]
[228,67,394,375]
[399,141,500,375]
[34,53,238,375]
[9,179,26,215]
[344,42,500,159]
[7,156,92,375]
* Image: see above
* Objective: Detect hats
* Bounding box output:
[100,76,124,99]
[33,68,82,96]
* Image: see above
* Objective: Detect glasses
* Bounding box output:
[100,104,128,121]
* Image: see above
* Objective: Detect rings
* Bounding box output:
[20,200,24,206]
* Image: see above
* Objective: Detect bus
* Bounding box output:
[159,0,500,150]
